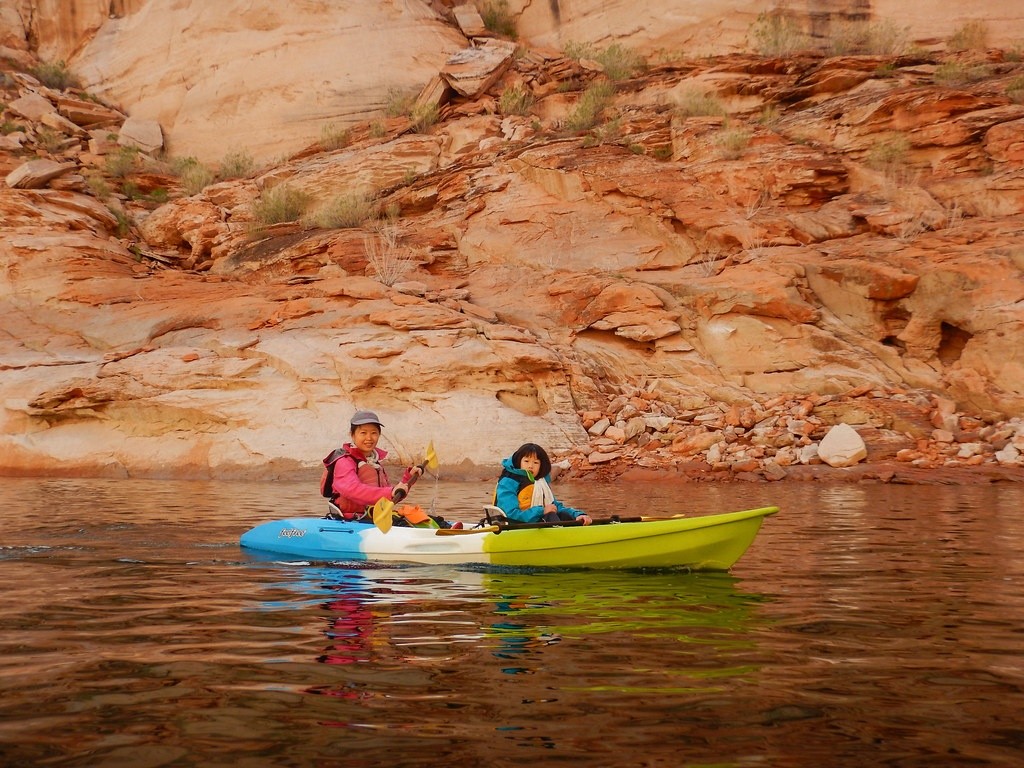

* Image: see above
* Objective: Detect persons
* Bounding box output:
[320,411,463,531]
[492,443,593,526]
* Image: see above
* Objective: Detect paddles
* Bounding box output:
[371,440,440,534]
[435,513,685,536]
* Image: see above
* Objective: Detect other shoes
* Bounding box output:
[449,522,464,529]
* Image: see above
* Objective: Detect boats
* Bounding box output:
[236,502,782,572]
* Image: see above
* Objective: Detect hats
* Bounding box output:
[350,411,385,426]
